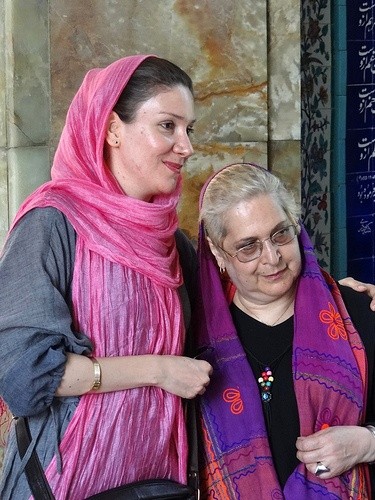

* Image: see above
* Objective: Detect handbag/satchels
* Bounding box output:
[83,476,195,500]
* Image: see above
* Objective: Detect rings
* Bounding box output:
[314,462,331,476]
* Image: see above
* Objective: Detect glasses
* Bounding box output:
[211,224,297,263]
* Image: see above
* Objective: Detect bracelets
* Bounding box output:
[366,424,375,440]
[89,356,103,392]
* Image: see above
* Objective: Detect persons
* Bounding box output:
[196,163,375,500]
[0,54,375,500]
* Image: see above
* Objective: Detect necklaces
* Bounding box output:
[238,280,298,327]
[242,332,293,403]
